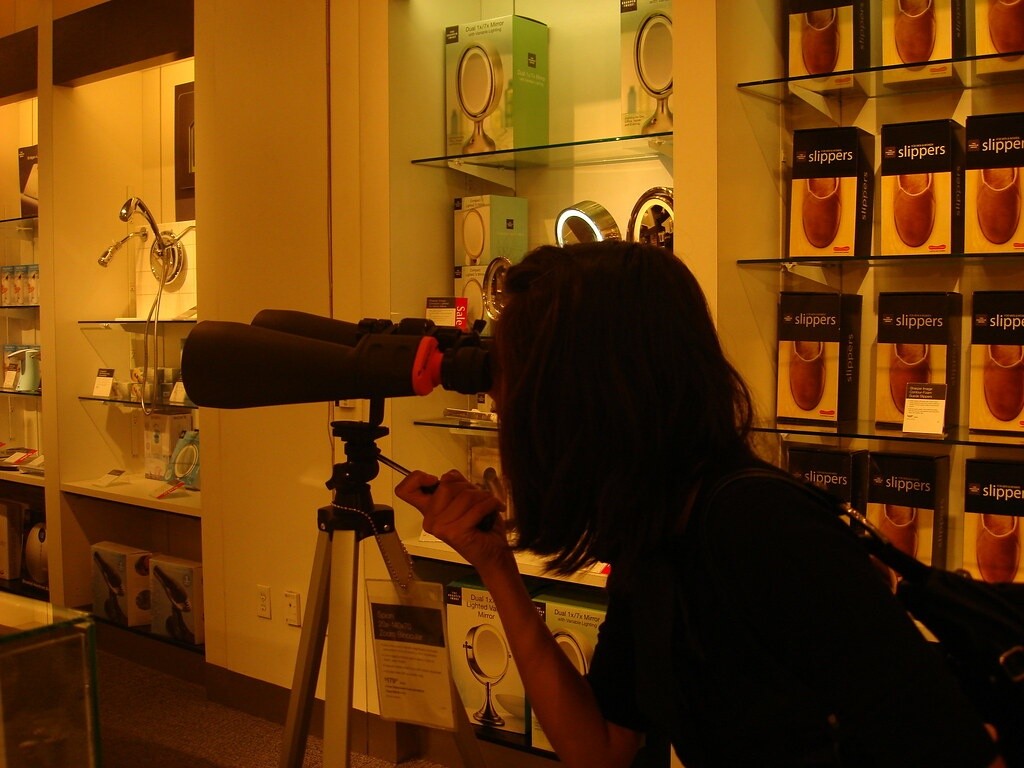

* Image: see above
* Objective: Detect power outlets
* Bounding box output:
[285,591,301,627]
[257,584,271,620]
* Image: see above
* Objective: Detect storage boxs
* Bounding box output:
[964,111,1024,255]
[787,443,868,517]
[445,15,550,171]
[0,498,204,646]
[777,290,862,422]
[969,289,1024,435]
[143,410,192,483]
[531,582,646,756]
[789,0,870,98]
[866,450,950,569]
[454,194,529,267]
[620,0,673,137]
[446,569,556,744]
[789,126,875,256]
[874,291,964,431]
[471,443,519,541]
[975,0,1024,84]
[881,119,965,256]
[883,0,966,92]
[963,457,1024,584]
[454,265,498,337]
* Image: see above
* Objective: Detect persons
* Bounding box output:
[394,240,1004,768]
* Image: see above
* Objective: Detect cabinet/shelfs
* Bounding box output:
[738,50,1024,448]
[410,131,673,432]
[60,319,205,689]
[0,214,50,603]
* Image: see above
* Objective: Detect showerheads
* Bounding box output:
[120,196,164,249]
[99,246,116,267]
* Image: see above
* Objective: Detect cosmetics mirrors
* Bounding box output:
[550,627,594,676]
[462,210,485,266]
[482,256,512,321]
[463,624,511,726]
[462,278,484,328]
[634,10,673,135]
[555,186,674,253]
[456,42,503,154]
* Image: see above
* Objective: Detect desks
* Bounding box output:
[0,591,102,768]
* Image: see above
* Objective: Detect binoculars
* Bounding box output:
[181,309,497,410]
